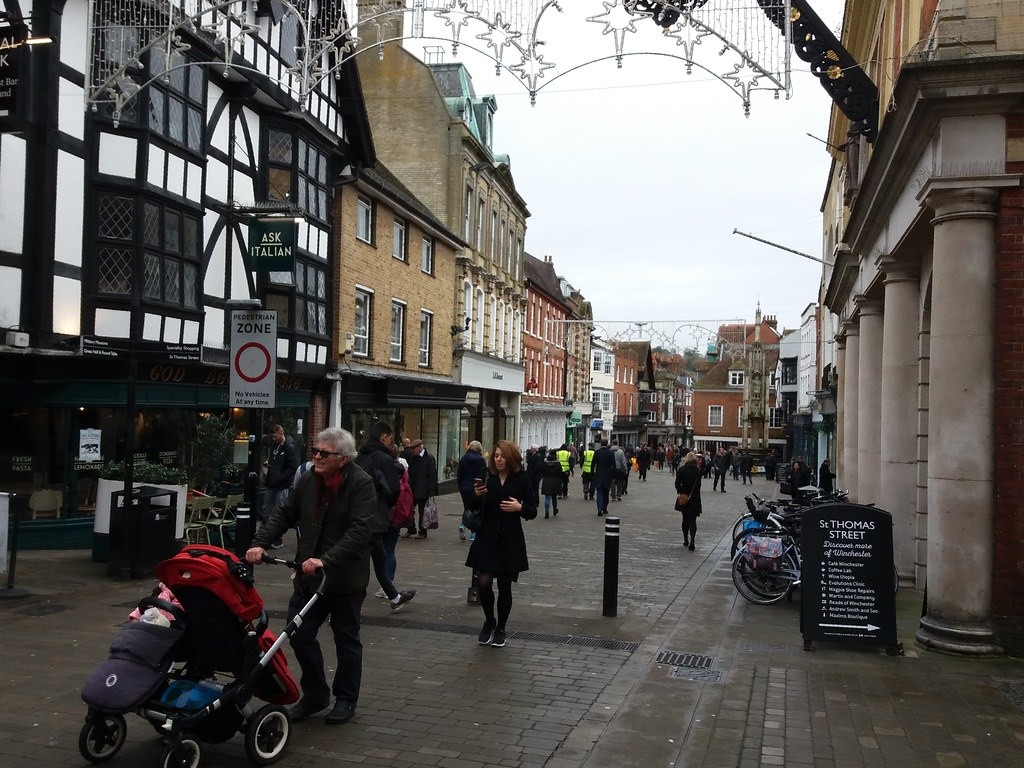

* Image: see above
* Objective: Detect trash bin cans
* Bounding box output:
[108,486,178,579]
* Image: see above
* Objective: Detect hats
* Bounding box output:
[409,439,422,448]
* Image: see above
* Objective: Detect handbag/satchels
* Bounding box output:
[462,472,489,533]
[780,481,793,495]
[746,536,783,571]
[634,463,640,472]
[678,493,688,506]
[388,469,414,529]
[422,495,439,529]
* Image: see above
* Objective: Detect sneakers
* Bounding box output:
[490,631,507,647]
[271,542,283,549]
[479,618,496,644]
[390,590,417,612]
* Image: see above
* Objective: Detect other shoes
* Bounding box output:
[721,490,726,492]
[469,537,474,541]
[459,528,466,540]
[713,486,716,490]
[325,700,355,725]
[415,534,427,539]
[288,700,330,723]
[374,588,389,600]
[584,489,627,517]
[688,545,695,550]
[545,494,566,519]
[684,540,689,546]
[402,532,416,538]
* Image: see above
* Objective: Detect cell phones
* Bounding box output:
[474,477,483,488]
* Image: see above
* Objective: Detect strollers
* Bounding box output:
[79,544,327,768]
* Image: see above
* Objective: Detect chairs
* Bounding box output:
[184,496,217,546]
[22,489,64,521]
[195,494,245,550]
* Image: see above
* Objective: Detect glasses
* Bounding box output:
[311,447,340,458]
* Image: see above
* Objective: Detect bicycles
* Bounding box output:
[730,486,899,607]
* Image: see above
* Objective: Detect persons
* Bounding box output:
[355,421,417,611]
[675,453,702,551]
[580,439,634,516]
[786,461,812,500]
[636,444,653,481]
[374,438,438,598]
[657,444,713,478]
[713,448,754,492]
[528,442,577,519]
[463,440,538,646]
[259,425,296,548]
[457,441,487,541]
[819,460,836,497]
[245,427,376,724]
[129,584,184,627]
[765,449,777,480]
